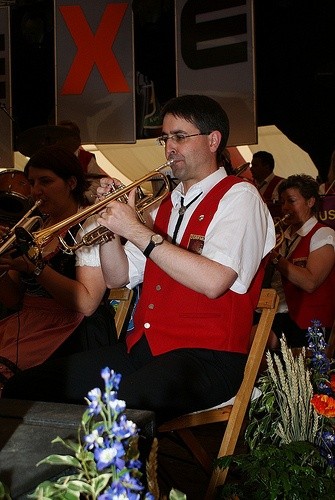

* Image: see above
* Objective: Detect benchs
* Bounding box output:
[0,399,155,500]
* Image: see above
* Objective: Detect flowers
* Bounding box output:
[23,316,335,500]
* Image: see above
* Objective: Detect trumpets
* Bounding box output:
[0,199,45,257]
[15,157,174,276]
[273,212,291,249]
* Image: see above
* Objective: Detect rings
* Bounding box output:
[106,207,112,213]
[101,212,107,219]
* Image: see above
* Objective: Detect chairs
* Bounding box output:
[105,288,134,340]
[157,288,280,500]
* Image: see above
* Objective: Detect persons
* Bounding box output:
[249,151,335,366]
[0,120,119,404]
[64,94,277,462]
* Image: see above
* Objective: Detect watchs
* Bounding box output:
[272,254,281,266]
[31,263,46,279]
[143,233,164,258]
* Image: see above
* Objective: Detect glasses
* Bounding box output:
[155,133,212,146]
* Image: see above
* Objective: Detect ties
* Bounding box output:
[258,181,267,192]
[172,190,203,245]
[284,234,300,258]
